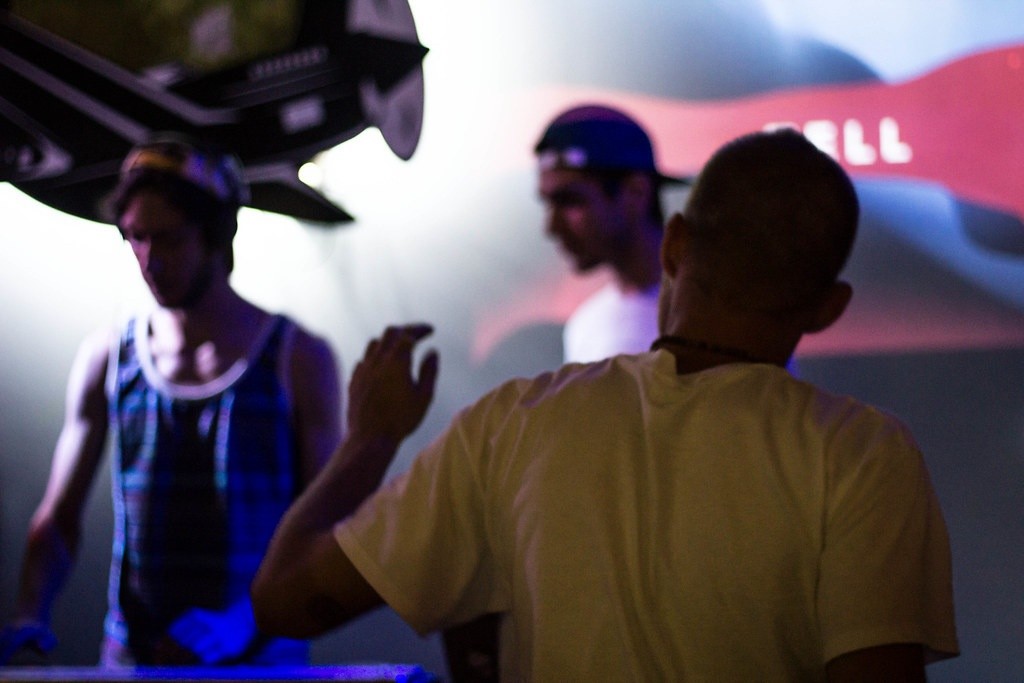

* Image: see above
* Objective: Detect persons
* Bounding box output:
[249,128,961,683]
[532,105,692,367]
[0,141,340,668]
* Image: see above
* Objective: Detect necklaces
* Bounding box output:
[648,333,768,366]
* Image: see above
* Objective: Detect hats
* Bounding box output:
[124,142,245,210]
[535,105,684,184]
